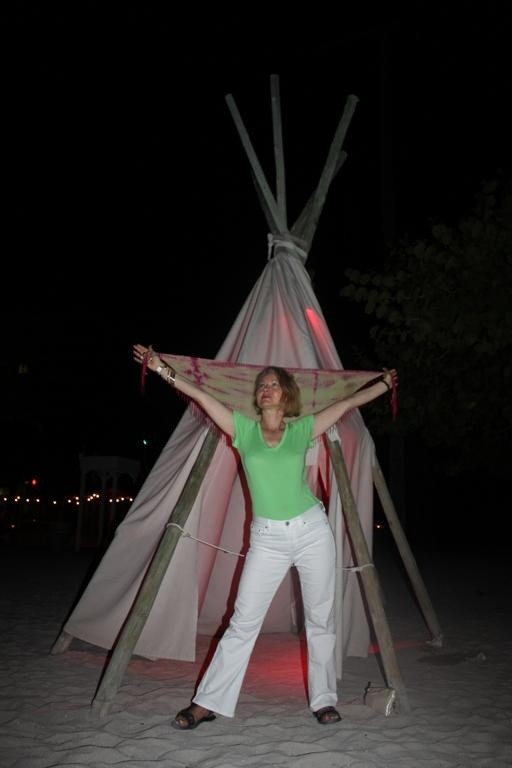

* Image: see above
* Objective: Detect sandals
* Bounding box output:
[313,705,341,724]
[172,703,216,729]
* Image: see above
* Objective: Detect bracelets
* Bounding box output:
[155,363,176,385]
[378,379,390,392]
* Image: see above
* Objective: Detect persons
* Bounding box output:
[131,343,398,730]
